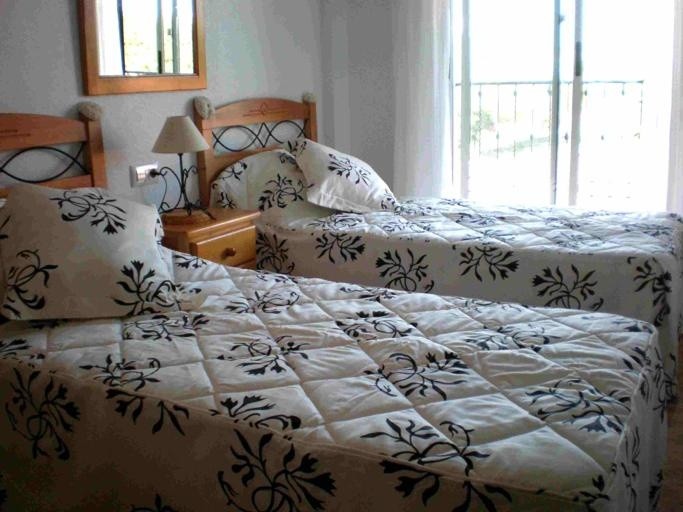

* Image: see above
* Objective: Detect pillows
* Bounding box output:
[0,180,184,323]
[286,137,401,217]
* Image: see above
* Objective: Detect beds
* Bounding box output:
[189,96,683,403]
[0,102,668,512]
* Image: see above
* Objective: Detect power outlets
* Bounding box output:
[128,161,163,187]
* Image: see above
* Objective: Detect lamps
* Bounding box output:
[150,114,216,222]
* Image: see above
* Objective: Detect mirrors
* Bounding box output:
[72,1,209,96]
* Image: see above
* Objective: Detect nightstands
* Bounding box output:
[150,202,261,274]
